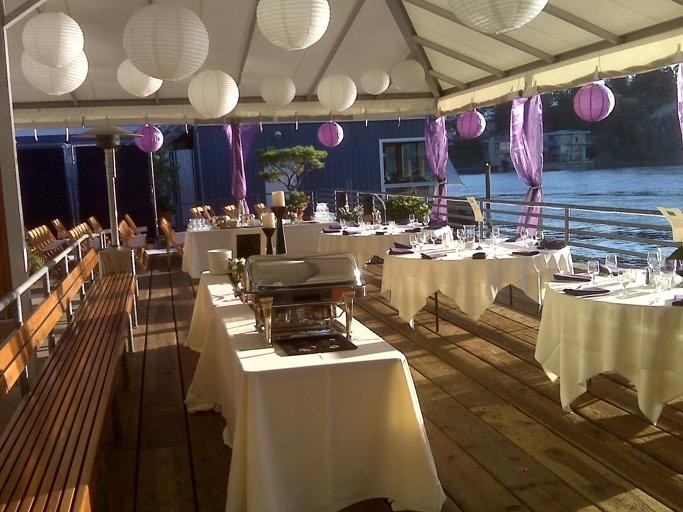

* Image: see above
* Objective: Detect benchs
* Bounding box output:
[0,247,138,512]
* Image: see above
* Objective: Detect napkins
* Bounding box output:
[553,272,590,282]
[672,295,682,305]
[420,252,447,260]
[598,265,625,275]
[563,285,611,299]
[388,247,414,255]
[512,249,539,256]
[394,241,412,248]
[472,252,488,260]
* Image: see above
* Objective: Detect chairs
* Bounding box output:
[188,203,268,221]
[25,190,184,271]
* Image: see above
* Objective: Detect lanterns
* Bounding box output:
[361,67,389,96]
[456,109,486,139]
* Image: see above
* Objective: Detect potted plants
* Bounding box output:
[255,144,328,220]
[379,195,432,225]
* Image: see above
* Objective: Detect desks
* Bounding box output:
[187,269,444,512]
[534,270,683,423]
[320,222,451,267]
[382,240,569,333]
[186,220,340,276]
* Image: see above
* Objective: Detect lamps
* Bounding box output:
[456,104,486,139]
[360,69,389,95]
